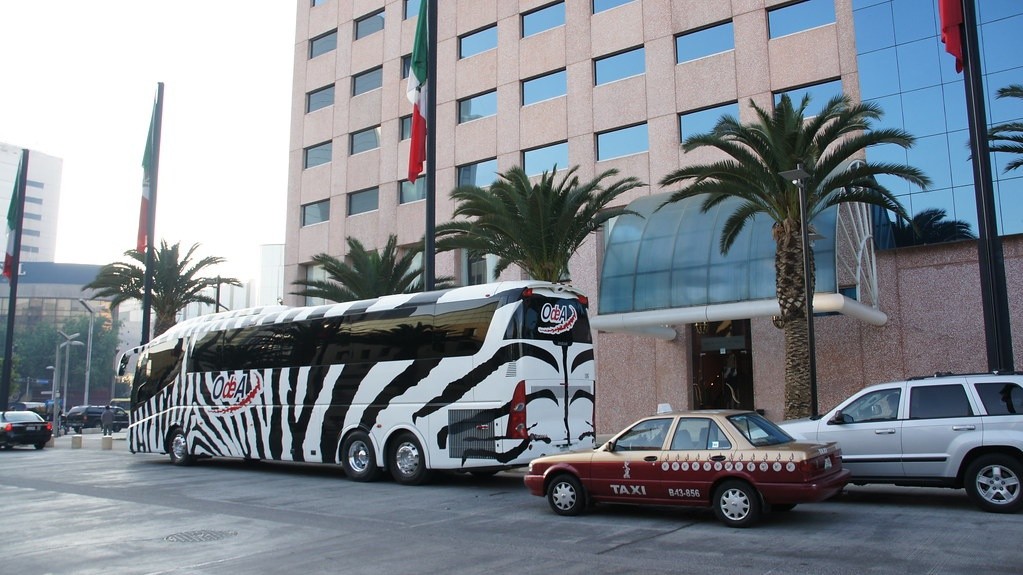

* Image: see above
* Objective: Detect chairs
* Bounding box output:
[673,428,693,447]
[696,428,715,448]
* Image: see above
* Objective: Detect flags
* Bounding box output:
[407,0,428,184]
[2,157,22,286]
[938,0,963,74]
[137,97,157,252]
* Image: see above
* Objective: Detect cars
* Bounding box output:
[523,410,853,530]
[0,411,53,451]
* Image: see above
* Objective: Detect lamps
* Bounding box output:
[694,322,709,336]
[772,316,784,330]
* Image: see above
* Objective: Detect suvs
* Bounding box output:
[743,371,1023,515]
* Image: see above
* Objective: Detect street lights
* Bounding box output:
[78,299,96,405]
[57,330,84,414]
[778,164,818,419]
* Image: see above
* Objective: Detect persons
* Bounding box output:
[659,425,670,439]
[101,406,113,435]
[887,393,900,417]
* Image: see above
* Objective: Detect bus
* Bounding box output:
[117,280,597,487]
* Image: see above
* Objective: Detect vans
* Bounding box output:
[67,405,128,433]
